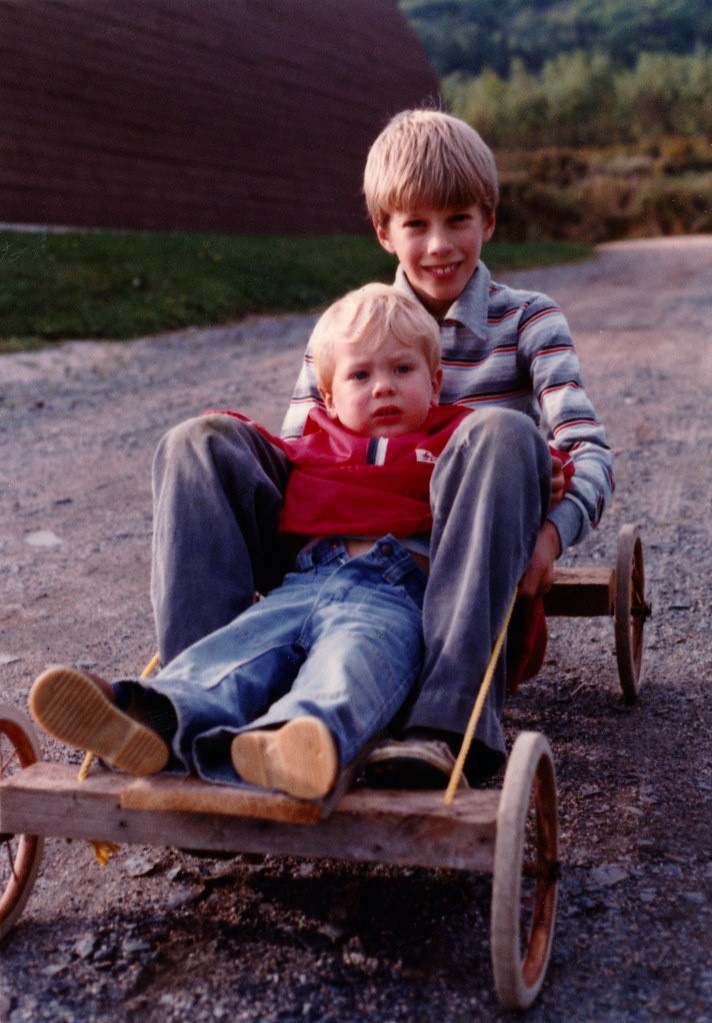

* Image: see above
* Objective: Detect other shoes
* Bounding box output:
[371,736,472,794]
[28,663,168,774]
[229,714,339,802]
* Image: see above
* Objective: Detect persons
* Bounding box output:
[28,283,575,799]
[150,108,615,791]
